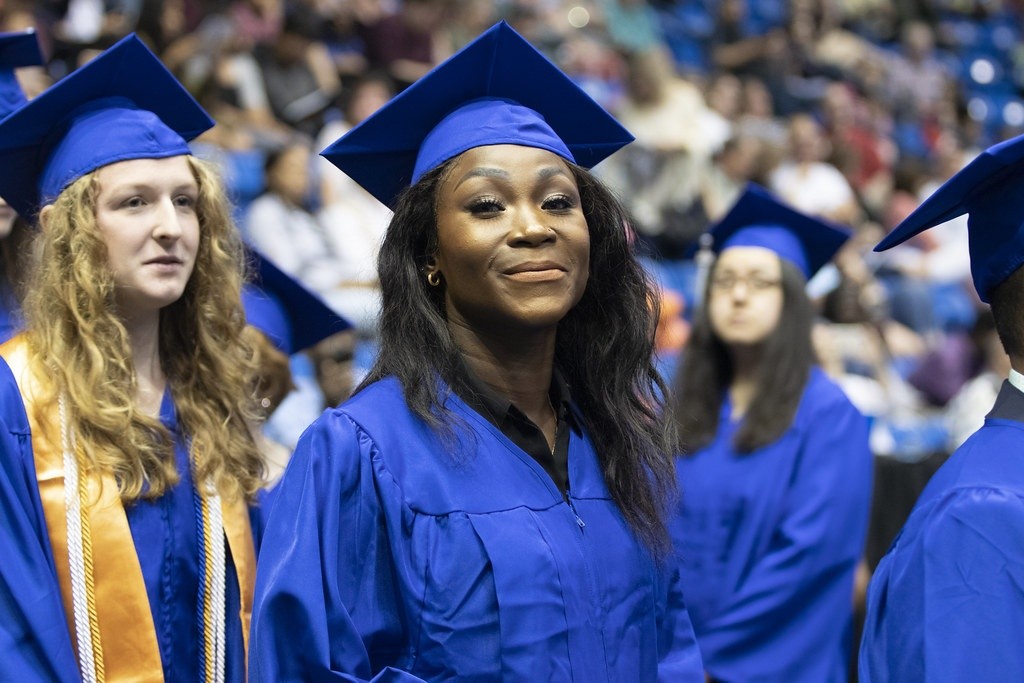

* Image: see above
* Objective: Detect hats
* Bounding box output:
[874,131,1023,302]
[234,237,355,359]
[1,32,219,231]
[704,183,850,284]
[0,28,44,120]
[321,17,636,214]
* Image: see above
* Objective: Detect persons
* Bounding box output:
[2,0,1023,550]
[2,32,271,683]
[249,27,706,682]
[651,186,871,682]
[853,134,1023,683]
